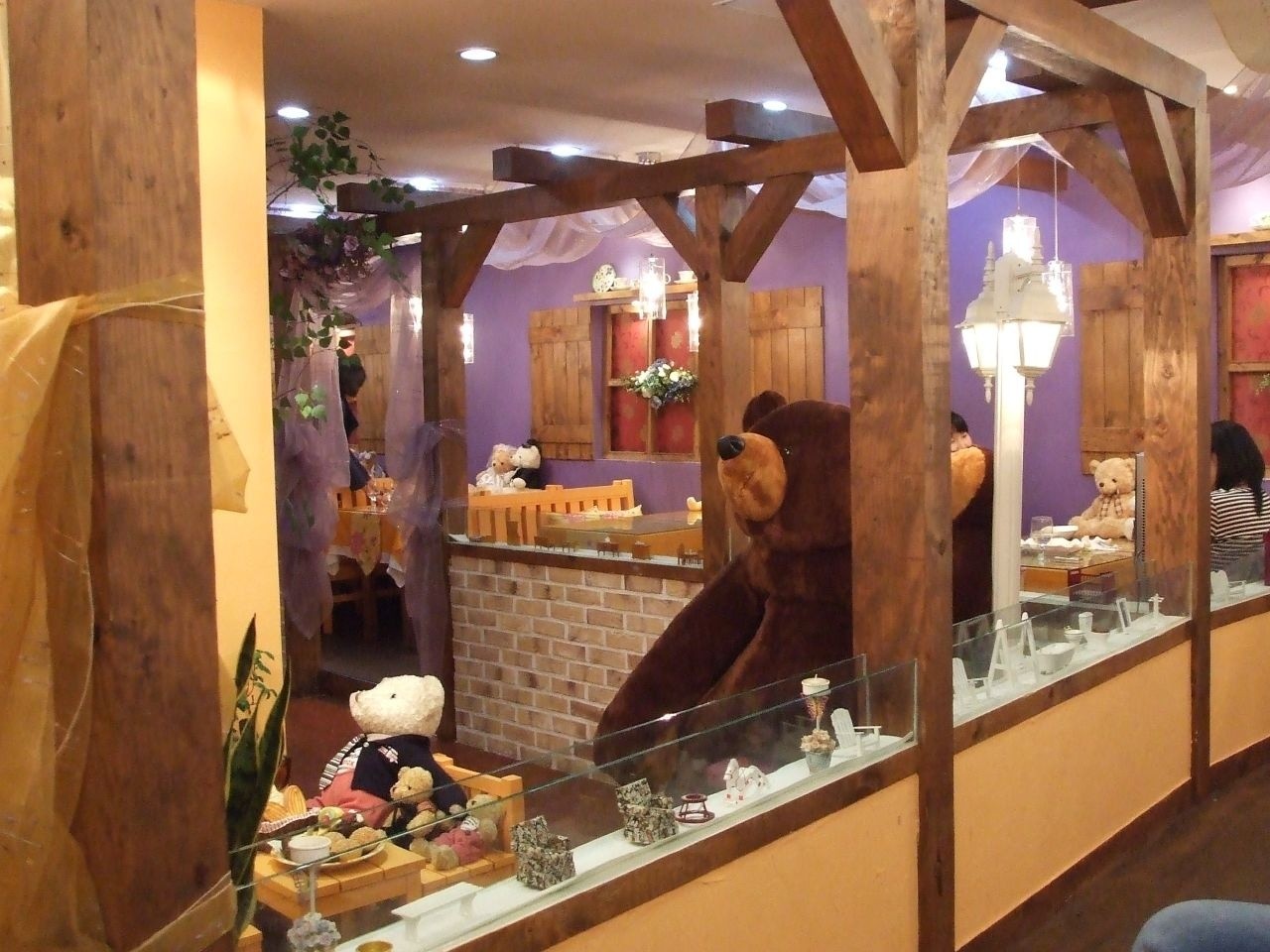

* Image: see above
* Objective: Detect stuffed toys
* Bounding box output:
[593,390,851,794]
[477,438,545,490]
[1068,456,1136,543]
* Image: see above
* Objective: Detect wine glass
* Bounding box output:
[1030,515,1053,563]
[365,480,396,511]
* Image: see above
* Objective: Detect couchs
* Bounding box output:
[614,777,680,846]
[508,814,578,891]
[329,474,394,629]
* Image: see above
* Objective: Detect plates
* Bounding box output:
[270,829,387,870]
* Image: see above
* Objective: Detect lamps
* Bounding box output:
[1001,145,1037,264]
[1041,156,1076,338]
[684,288,701,353]
[952,237,1068,656]
[636,250,668,321]
[459,313,474,364]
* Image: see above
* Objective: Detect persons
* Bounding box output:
[338,350,371,491]
[950,411,993,624]
[1210,420,1269,582]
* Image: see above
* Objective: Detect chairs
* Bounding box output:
[694,547,704,565]
[830,707,884,761]
[953,657,995,714]
[561,539,578,554]
[1211,569,1247,605]
[675,541,692,566]
[358,751,525,934]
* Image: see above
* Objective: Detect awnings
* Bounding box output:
[305,676,506,871]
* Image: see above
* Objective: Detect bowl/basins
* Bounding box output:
[1052,526,1079,540]
[1020,537,1084,556]
[287,836,332,864]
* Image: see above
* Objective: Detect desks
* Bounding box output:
[250,833,426,932]
[539,509,704,560]
[595,541,620,557]
[684,551,698,565]
[1019,532,1140,602]
[326,479,544,649]
[631,542,652,560]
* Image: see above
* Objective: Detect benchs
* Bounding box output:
[391,880,484,944]
[469,477,636,548]
[534,535,555,551]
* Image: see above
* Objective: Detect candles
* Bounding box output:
[800,674,832,697]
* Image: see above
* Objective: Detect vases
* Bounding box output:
[805,749,836,774]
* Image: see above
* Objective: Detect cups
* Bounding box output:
[493,472,504,494]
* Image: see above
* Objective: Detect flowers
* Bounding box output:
[287,912,343,952]
[797,691,837,755]
[617,358,700,412]
